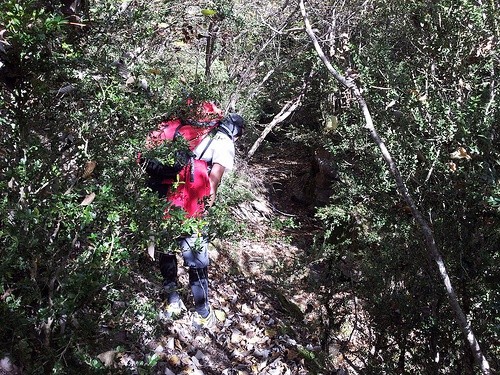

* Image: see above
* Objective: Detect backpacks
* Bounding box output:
[147,125,195,202]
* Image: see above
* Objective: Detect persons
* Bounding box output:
[137,115,246,329]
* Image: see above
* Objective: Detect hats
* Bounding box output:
[222,114,246,135]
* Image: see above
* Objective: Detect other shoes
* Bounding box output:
[167,305,181,317]
[200,306,212,320]
